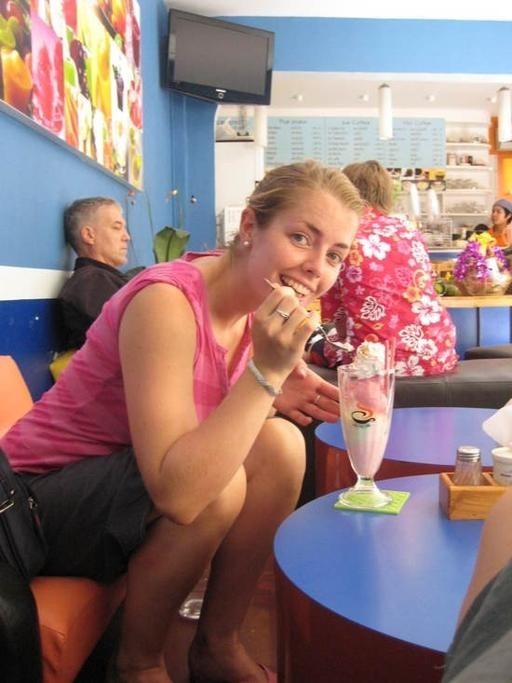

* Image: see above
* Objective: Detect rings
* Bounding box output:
[276,310,290,320]
[313,394,320,404]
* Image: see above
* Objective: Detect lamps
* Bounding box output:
[379,84,392,139]
[497,88,512,142]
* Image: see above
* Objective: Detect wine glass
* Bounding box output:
[338,362,392,511]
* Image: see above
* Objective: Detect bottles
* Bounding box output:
[453,448,483,487]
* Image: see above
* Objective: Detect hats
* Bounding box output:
[493,199,512,213]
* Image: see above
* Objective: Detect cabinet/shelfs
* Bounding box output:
[443,120,494,216]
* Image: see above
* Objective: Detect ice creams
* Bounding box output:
[336,342,396,477]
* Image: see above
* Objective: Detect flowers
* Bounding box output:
[453,240,509,283]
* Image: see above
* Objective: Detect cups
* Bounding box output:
[489,447,512,484]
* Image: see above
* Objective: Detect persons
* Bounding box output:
[1,156,376,678]
[480,197,511,257]
[436,483,511,682]
[46,195,134,357]
[294,156,460,379]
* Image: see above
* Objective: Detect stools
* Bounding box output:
[465,344,512,360]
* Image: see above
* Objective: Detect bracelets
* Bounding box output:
[246,358,286,396]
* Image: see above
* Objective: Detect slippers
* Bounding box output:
[189,660,279,683]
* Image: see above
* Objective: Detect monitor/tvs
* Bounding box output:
[164,8,275,105]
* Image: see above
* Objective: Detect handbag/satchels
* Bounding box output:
[1,449,48,577]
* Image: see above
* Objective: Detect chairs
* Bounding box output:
[0,355,126,683]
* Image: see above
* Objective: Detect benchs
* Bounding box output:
[274,358,512,511]
